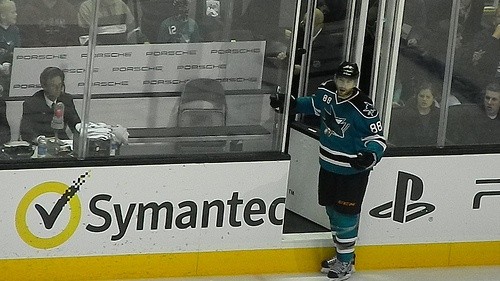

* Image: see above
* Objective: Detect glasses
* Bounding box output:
[300,21,305,27]
[46,82,62,89]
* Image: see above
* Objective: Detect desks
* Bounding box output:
[120,124,271,156]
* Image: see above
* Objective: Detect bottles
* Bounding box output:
[37,135,47,160]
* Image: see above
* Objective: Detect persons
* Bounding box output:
[278,8,342,85]
[0,0,500,100]
[269,62,388,280]
[19,67,81,148]
[444,83,500,145]
[387,82,441,146]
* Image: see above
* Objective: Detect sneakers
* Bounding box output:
[327,260,353,280]
[321,254,356,273]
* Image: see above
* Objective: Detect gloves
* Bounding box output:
[349,152,377,171]
[269,92,296,114]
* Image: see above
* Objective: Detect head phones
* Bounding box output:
[40,67,65,87]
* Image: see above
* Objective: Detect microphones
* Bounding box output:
[63,83,65,92]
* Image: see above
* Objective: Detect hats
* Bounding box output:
[336,62,359,79]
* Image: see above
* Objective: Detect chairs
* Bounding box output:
[176,78,227,153]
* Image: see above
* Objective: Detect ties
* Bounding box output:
[52,103,55,112]
